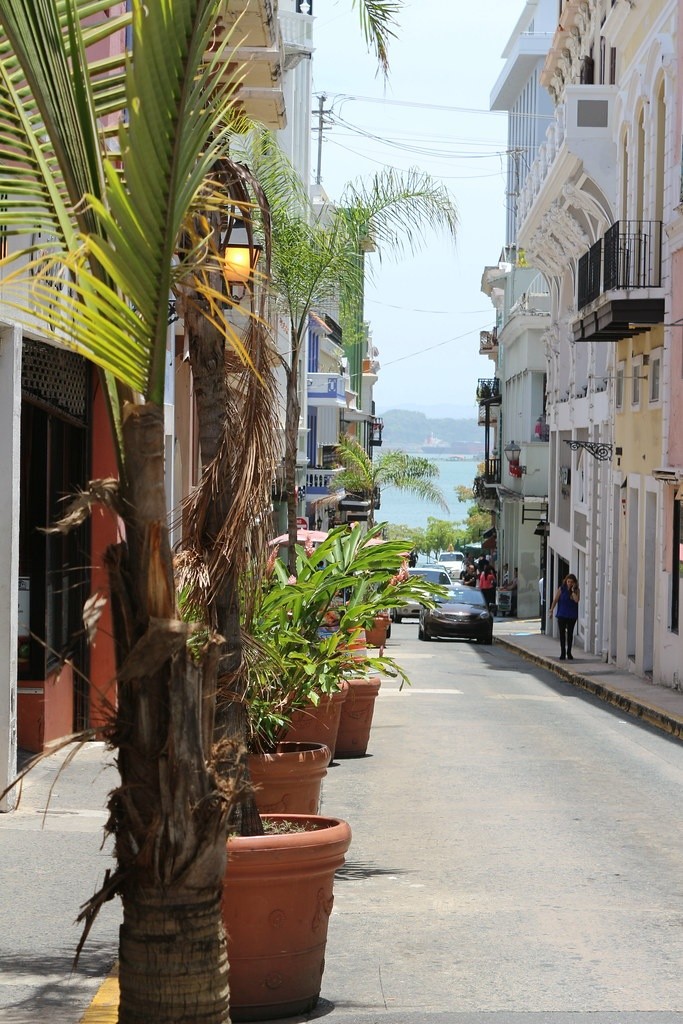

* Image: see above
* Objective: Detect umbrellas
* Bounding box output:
[356,533,389,548]
[266,526,339,548]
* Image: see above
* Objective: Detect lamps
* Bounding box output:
[503,437,527,477]
[212,210,265,300]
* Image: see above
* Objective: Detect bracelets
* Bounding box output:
[572,591,574,593]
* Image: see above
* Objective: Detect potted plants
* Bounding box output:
[201,507,452,1017]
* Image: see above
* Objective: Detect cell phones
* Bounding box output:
[571,585,573,588]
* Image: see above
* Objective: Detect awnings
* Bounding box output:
[483,527,496,538]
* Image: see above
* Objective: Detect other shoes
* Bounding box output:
[560,651,566,660]
[567,650,573,660]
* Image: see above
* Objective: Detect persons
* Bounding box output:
[547,573,580,661]
[408,549,418,568]
[461,552,518,616]
[537,576,546,632]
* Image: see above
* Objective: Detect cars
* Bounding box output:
[438,551,467,582]
[416,585,498,644]
[423,564,452,579]
[378,567,455,628]
